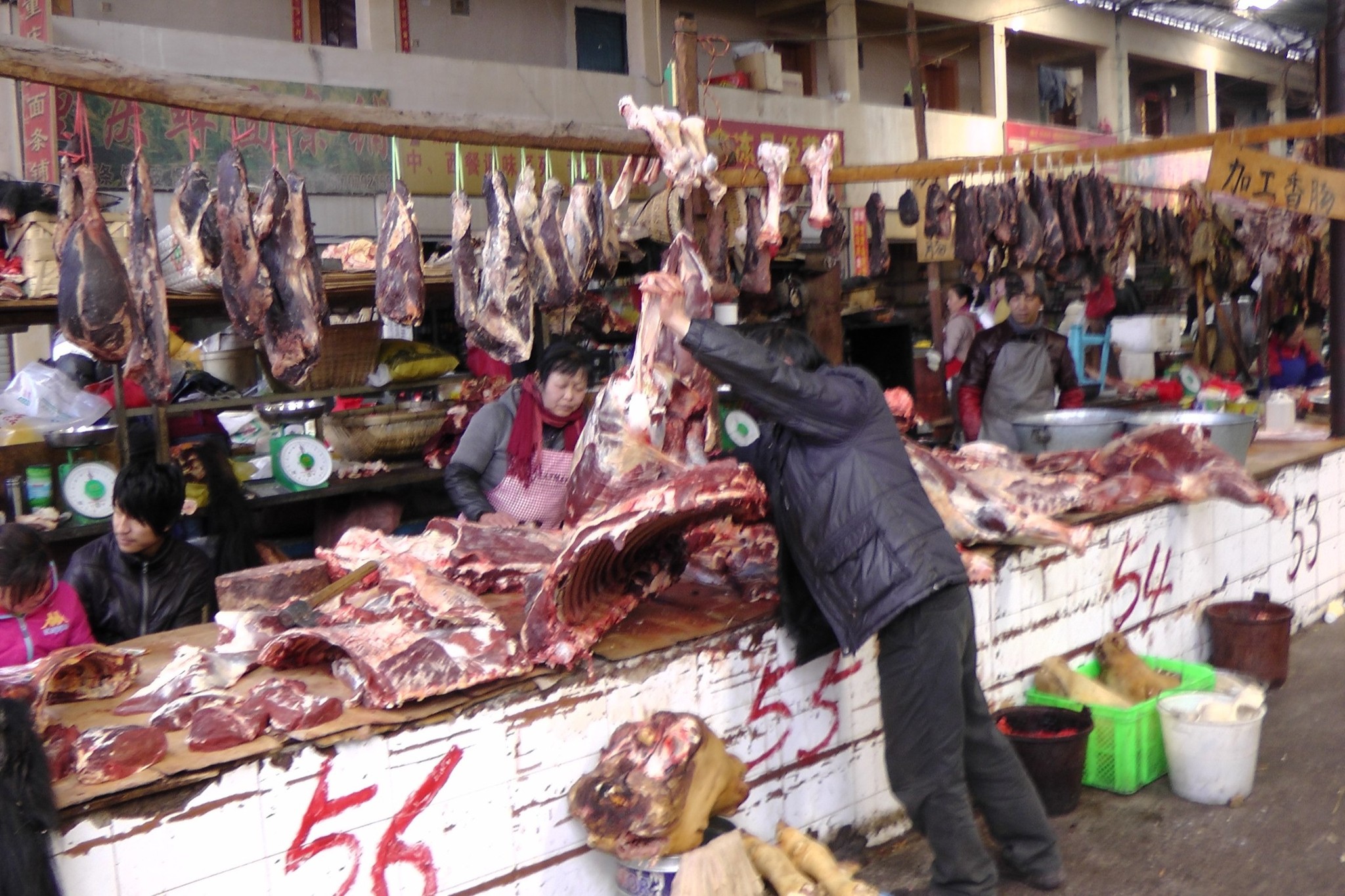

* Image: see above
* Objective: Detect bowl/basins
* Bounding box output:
[196,332,259,396]
[1122,409,1256,469]
[1012,407,1131,454]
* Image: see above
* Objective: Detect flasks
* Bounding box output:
[3,475,23,520]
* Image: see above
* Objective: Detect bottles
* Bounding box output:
[1265,392,1294,436]
[26,464,56,514]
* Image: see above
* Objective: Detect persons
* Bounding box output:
[874,267,1136,458]
[450,341,597,530]
[47,323,241,406]
[1307,100,1322,141]
[0,461,216,896]
[1260,315,1325,388]
[638,270,1071,896]
[462,328,514,377]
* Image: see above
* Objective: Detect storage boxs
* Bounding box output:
[703,51,804,96]
[1023,655,1213,794]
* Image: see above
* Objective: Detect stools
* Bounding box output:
[1068,323,1112,393]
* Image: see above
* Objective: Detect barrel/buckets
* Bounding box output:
[1155,685,1269,805]
[988,703,1097,816]
[1205,591,1294,691]
[617,814,736,896]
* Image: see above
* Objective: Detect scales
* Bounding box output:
[1156,351,1201,396]
[717,387,760,450]
[43,425,117,525]
[255,400,332,491]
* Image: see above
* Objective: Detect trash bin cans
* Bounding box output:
[1203,590,1296,694]
[993,703,1093,816]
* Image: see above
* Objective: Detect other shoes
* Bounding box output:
[889,852,1067,896]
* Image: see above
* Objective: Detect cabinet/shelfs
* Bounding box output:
[0,373,474,542]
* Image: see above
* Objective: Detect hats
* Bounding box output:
[1004,272,1048,301]
